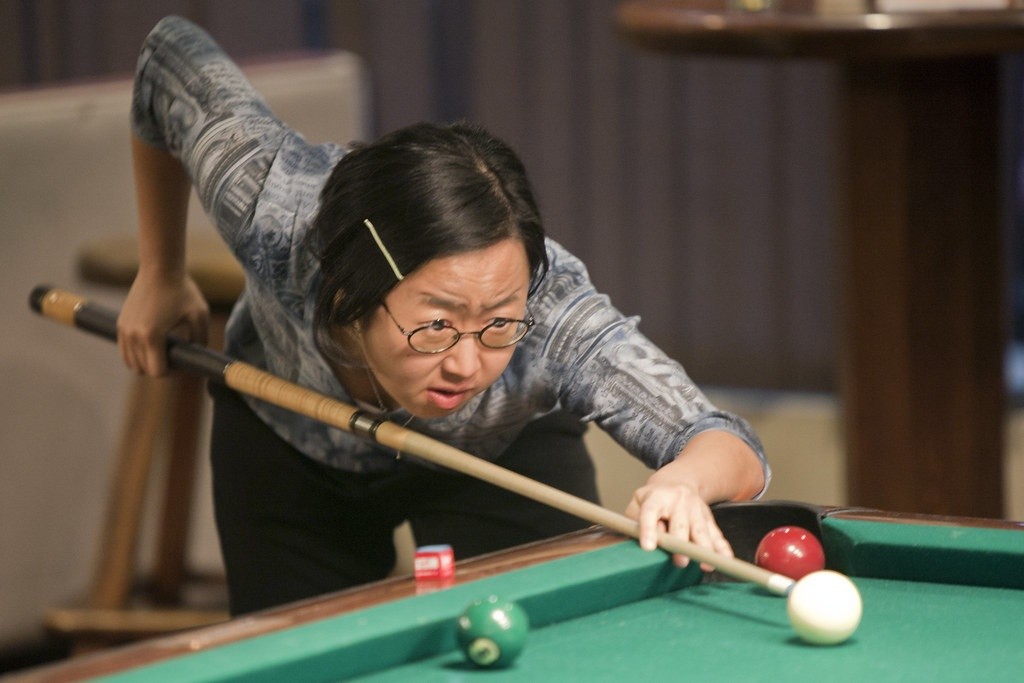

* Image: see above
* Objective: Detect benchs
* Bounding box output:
[70,231,249,656]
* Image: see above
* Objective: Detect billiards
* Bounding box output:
[756,525,828,582]
[788,568,864,647]
[455,594,529,665]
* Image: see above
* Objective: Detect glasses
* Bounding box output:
[377,293,536,354]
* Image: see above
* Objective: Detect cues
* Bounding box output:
[28,284,794,594]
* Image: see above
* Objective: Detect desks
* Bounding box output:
[0,500,1024,683]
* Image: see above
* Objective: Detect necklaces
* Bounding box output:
[361,356,415,461]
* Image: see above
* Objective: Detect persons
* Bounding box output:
[117,15,774,624]
